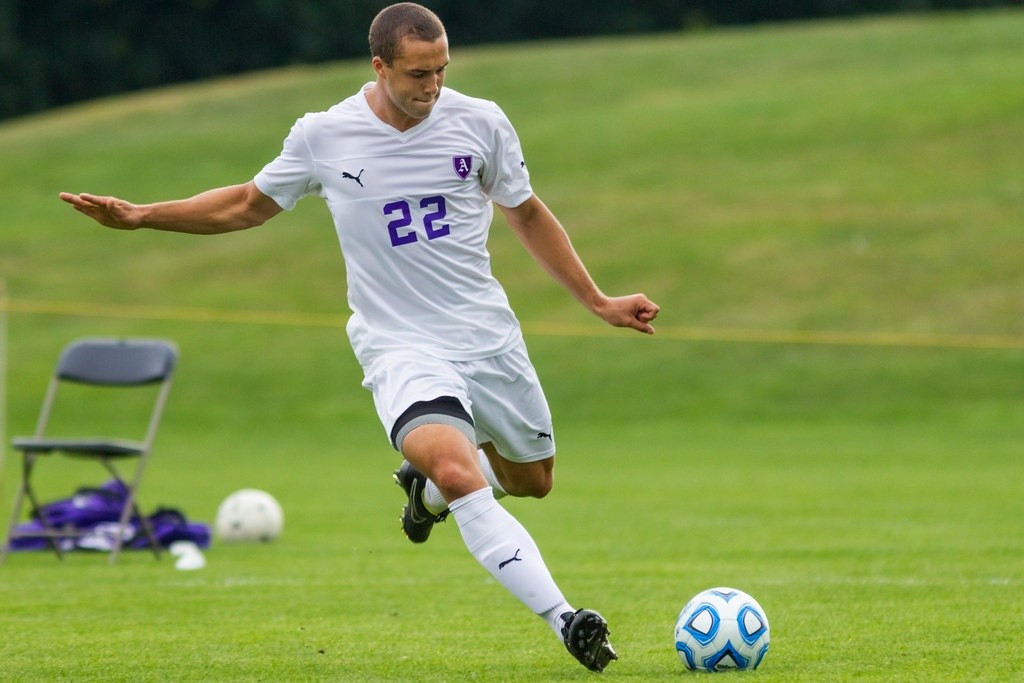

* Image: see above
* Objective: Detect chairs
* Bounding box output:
[4,336,177,564]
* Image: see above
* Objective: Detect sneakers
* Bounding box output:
[558,609,619,672]
[392,457,447,544]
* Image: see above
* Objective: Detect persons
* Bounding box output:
[57,2,663,674]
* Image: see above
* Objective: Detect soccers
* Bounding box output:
[674,587,772,671]
[217,488,283,542]
